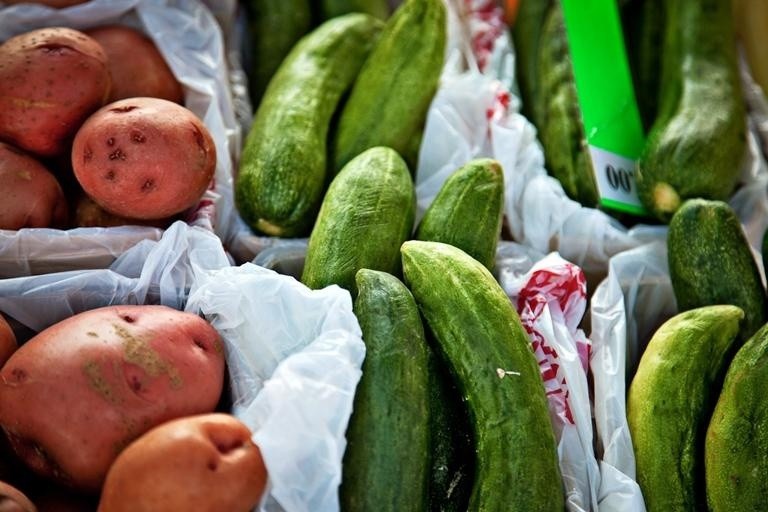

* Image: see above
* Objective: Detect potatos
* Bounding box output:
[1,300,267,512]
[1,22,217,229]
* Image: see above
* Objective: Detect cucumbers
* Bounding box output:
[232,0,768,511]
